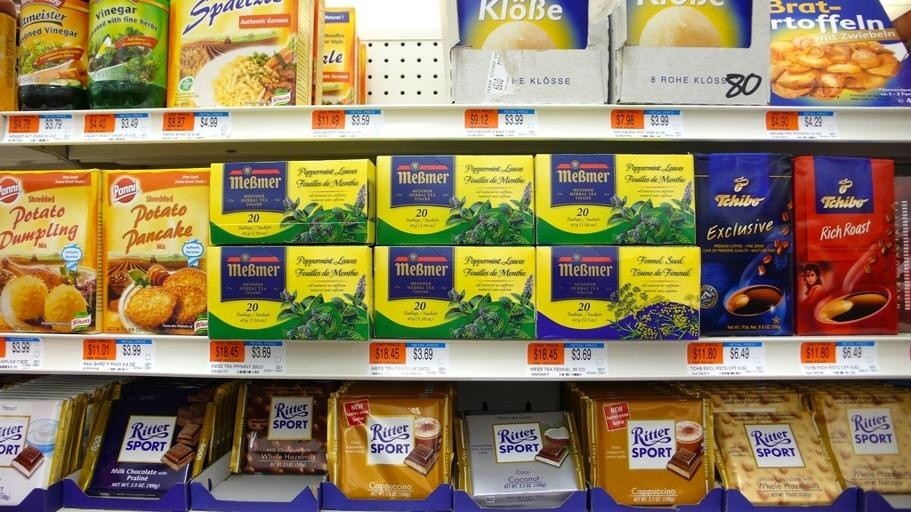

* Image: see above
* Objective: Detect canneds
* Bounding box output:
[89,0,170,109]
[18,0,88,109]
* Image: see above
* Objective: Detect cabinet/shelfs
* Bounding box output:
[0,102,911,383]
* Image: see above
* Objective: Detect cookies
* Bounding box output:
[773,35,900,100]
[126,269,208,332]
[8,276,87,332]
